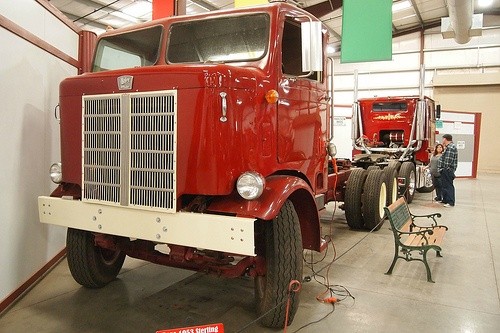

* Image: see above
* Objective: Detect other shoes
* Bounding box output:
[434,196,443,201]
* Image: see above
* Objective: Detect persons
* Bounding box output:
[423,144,444,201]
[438,134,458,208]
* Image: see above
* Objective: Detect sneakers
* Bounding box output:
[443,203,455,208]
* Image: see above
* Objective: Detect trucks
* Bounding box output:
[351,94,441,205]
[38,0,417,328]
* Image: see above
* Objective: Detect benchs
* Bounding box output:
[383,194,449,283]
